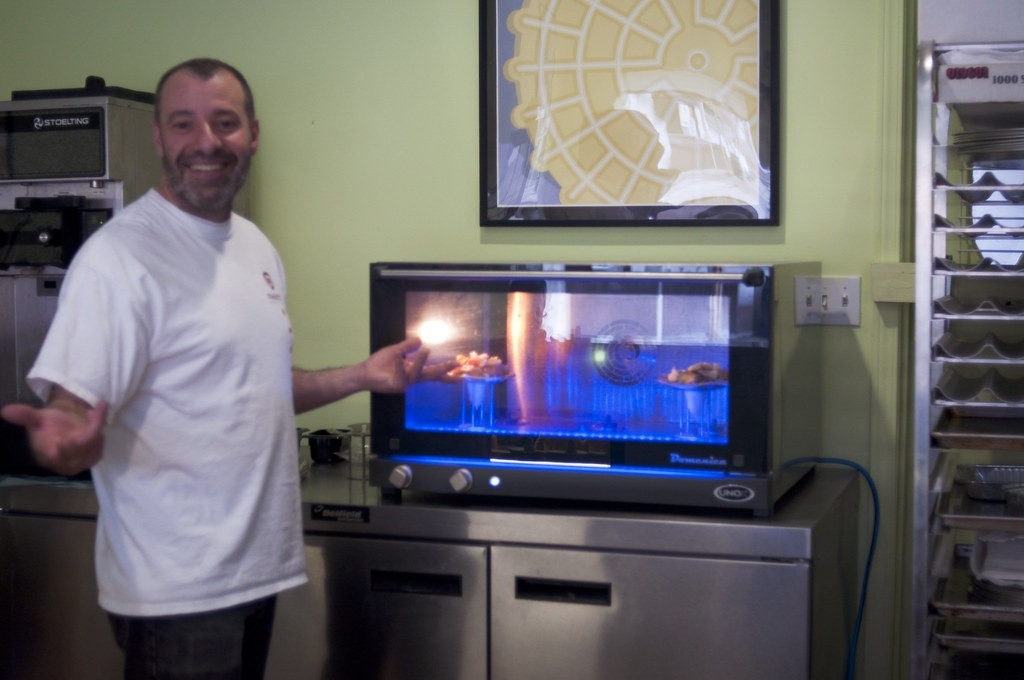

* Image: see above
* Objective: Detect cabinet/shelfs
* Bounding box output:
[912,41,1024,680]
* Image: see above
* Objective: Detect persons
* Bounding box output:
[2,56,458,680]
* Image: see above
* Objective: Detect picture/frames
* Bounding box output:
[479,0,779,228]
